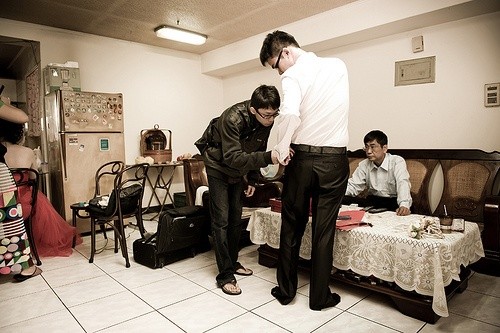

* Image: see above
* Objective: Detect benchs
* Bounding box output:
[346,149,500,278]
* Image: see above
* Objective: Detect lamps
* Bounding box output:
[153,25,209,45]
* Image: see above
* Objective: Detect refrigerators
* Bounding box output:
[42,89,126,235]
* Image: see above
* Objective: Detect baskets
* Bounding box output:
[141,129,172,164]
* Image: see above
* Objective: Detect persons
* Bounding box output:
[344,128,413,216]
[0,96,43,281]
[0,118,83,257]
[260,30,349,310]
[194,84,294,295]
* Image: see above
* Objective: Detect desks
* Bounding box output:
[126,161,185,221]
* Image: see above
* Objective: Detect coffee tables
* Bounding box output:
[246,206,485,326]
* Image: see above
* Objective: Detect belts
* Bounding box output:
[290,144,347,154]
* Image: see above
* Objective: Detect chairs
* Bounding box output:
[9,168,42,268]
[183,155,283,249]
[71,161,150,268]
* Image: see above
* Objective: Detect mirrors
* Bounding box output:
[0,35,47,161]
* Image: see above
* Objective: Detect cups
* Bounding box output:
[440,215,453,234]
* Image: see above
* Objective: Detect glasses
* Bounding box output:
[363,145,383,151]
[274,46,286,71]
[255,109,279,119]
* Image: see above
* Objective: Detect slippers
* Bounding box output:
[12,266,42,281]
[234,266,253,276]
[221,278,241,295]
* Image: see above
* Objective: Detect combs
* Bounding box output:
[0,85,5,95]
